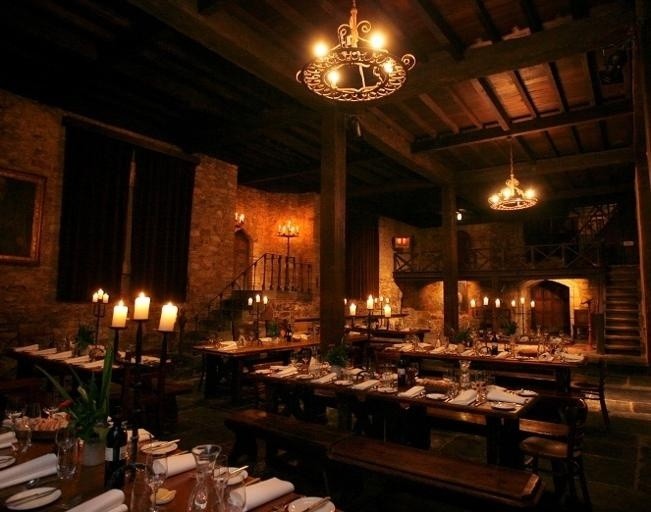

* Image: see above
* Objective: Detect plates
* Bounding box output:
[257,341,581,409]
[6,487,62,510]
[206,467,247,485]
[284,497,335,511]
[124,428,156,443]
[140,441,178,454]
[5,419,66,443]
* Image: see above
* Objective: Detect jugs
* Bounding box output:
[186,444,224,511]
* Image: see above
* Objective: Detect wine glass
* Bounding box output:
[146,452,168,509]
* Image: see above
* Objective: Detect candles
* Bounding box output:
[471,296,535,308]
[91,288,109,304]
[111,292,179,332]
[344,293,391,318]
[248,294,268,306]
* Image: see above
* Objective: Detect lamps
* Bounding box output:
[487,136,540,212]
[277,220,300,292]
[295,0,417,103]
[233,212,245,226]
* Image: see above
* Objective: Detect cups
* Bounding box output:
[115,464,156,511]
[10,423,33,457]
[222,484,247,511]
[57,440,84,484]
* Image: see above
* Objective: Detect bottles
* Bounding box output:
[103,416,130,492]
